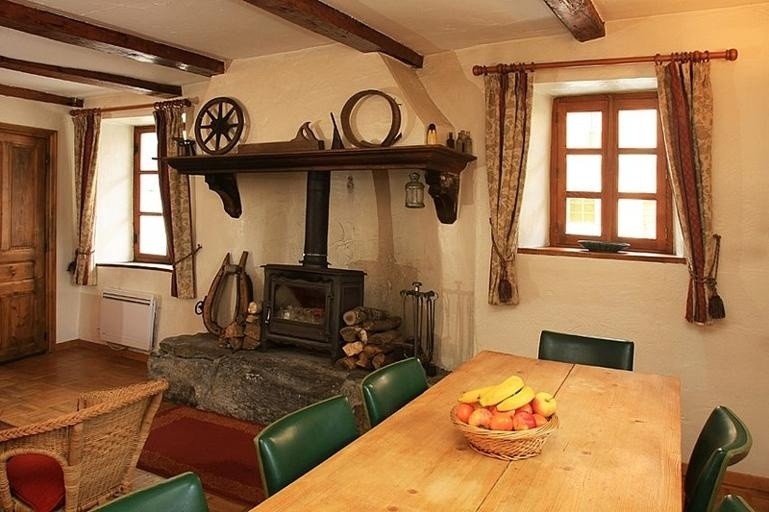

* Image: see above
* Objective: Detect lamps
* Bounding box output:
[405,171,425,208]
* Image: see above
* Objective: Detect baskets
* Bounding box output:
[450,403,559,461]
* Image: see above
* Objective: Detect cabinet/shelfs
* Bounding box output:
[152,142,478,224]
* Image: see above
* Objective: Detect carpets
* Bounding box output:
[136,405,269,507]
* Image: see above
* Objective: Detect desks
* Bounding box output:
[248,350,682,511]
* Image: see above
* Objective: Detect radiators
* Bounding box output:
[99,288,157,352]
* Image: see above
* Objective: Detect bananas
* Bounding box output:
[457,375,536,411]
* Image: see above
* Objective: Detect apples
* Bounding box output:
[457,393,557,430]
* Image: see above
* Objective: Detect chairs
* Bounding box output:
[0,378,170,509]
[537,329,634,371]
[88,471,209,511]
[715,492,756,511]
[253,393,360,499]
[684,404,752,511]
[360,356,429,426]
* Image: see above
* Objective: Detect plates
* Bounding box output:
[576,240,630,253]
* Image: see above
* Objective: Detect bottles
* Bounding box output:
[446,129,473,155]
[426,121,438,146]
